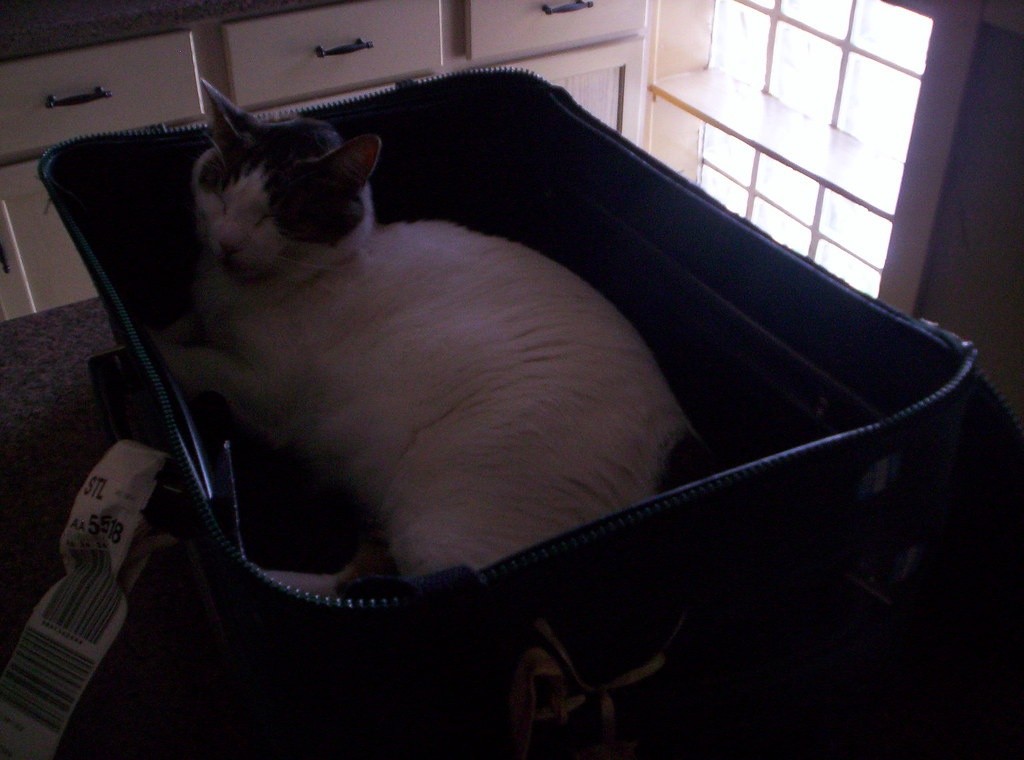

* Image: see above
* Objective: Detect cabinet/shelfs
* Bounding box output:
[0,0,644,321]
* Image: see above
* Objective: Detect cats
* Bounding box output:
[157,78,695,602]
